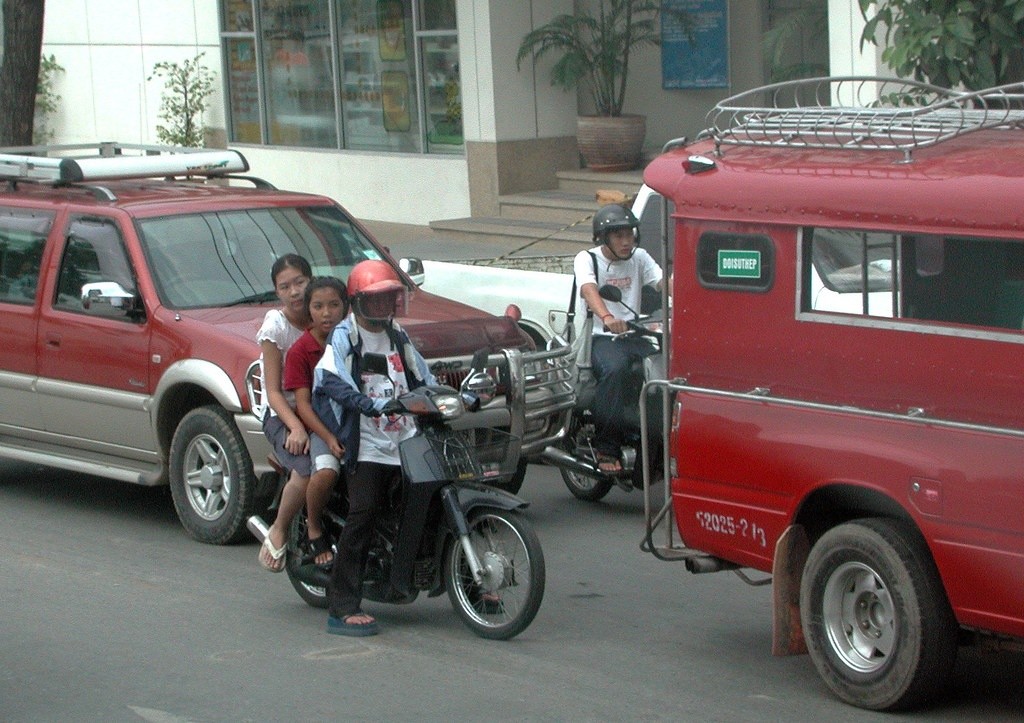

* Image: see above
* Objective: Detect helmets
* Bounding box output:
[346,259,404,300]
[592,205,640,244]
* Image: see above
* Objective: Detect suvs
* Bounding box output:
[0,142,579,545]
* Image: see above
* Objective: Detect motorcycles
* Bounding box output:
[538,283,670,501]
[243,350,546,643]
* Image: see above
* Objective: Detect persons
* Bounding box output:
[8,237,69,306]
[222,234,273,286]
[312,260,505,636]
[284,276,350,566]
[258,253,314,572]
[574,204,673,474]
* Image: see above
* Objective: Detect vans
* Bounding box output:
[640,76,1024,710]
[332,33,381,123]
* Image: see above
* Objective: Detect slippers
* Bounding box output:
[326,607,378,637]
[305,532,335,569]
[596,449,622,476]
[457,581,503,615]
[258,521,289,572]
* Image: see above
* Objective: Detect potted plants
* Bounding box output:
[512,0,698,174]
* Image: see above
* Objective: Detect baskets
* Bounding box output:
[427,427,522,481]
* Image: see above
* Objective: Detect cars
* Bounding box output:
[627,183,901,320]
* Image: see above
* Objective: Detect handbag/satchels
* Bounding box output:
[551,312,597,407]
